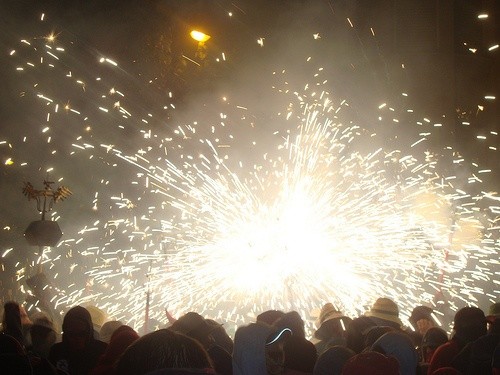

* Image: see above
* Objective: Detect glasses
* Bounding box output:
[65,330,91,338]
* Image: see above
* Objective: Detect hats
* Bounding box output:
[314,303,352,340]
[97,318,126,343]
[363,297,405,326]
[266,327,293,349]
[23,316,59,335]
[87,305,107,325]
[486,302,500,321]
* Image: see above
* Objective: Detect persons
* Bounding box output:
[0,298,500,375]
[0,257,22,316]
